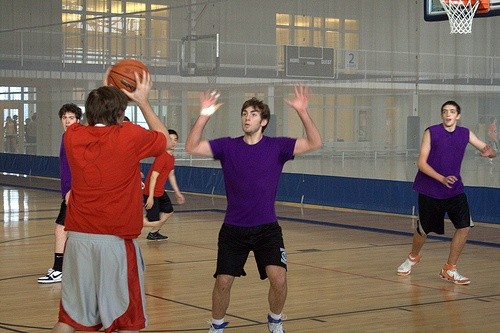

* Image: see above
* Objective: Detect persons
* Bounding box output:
[184,82,323,333]
[3,111,37,152]
[487,113,500,165]
[396,102,496,287]
[35,58,184,333]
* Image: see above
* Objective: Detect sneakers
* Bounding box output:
[208,322,229,333]
[37,268,63,283]
[396,254,421,276]
[146,229,169,241]
[438,266,471,285]
[266,320,286,333]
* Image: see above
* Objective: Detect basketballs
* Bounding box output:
[106,60,149,98]
